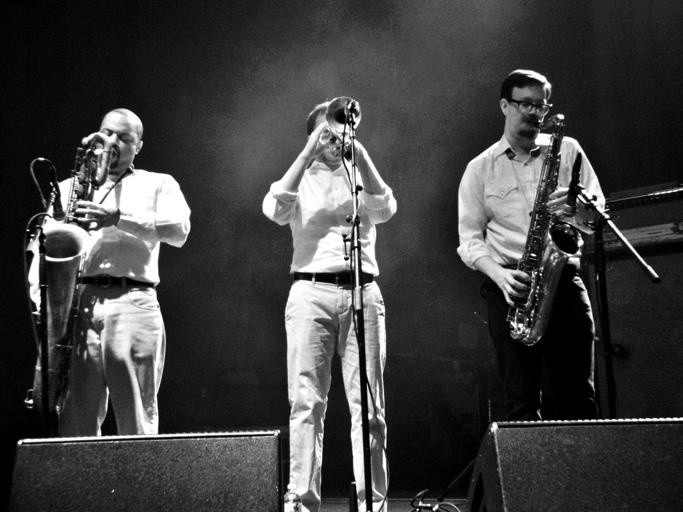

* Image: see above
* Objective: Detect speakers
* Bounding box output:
[10,428,284,512]
[463,417,683,512]
[580,235,682,417]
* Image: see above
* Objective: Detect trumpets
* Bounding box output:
[319,97,361,156]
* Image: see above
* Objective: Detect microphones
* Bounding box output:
[42,164,66,224]
[561,151,582,218]
[343,100,357,115]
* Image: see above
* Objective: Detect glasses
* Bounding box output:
[505,96,554,117]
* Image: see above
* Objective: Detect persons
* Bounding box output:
[261,98,398,511]
[26,106,189,436]
[454,69,601,418]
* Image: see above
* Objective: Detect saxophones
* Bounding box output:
[25,132,113,421]
[505,113,583,346]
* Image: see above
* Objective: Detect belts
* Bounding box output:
[292,270,375,287]
[75,274,156,290]
